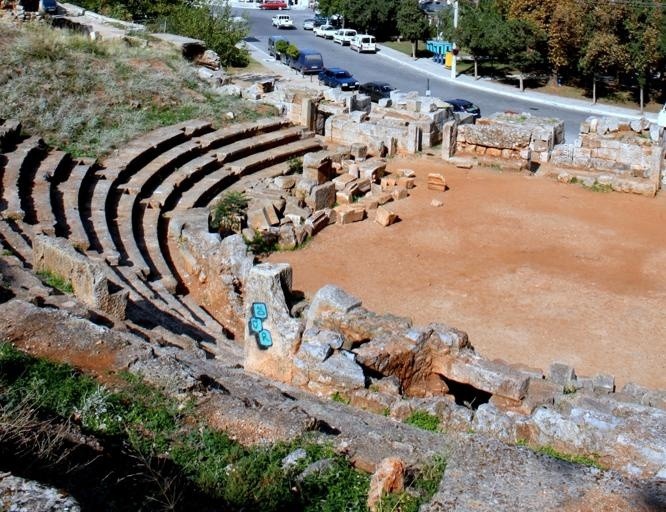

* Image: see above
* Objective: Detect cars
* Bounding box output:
[259,0,317,30]
[444,99,480,119]
[358,81,396,102]
[317,67,360,91]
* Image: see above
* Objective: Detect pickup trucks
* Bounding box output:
[313,25,336,40]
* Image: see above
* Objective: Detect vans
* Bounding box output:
[288,48,324,74]
[333,28,377,53]
